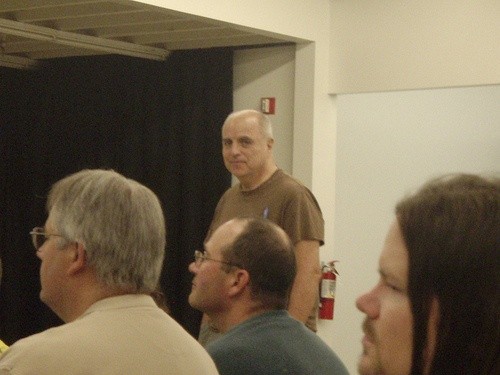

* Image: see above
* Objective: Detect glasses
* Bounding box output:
[29,227,74,252]
[194,250,246,272]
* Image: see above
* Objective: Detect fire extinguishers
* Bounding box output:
[318,259,341,320]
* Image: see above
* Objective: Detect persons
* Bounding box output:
[198,110,325,347]
[0,169,219,375]
[188,215,351,375]
[355,173,500,375]
